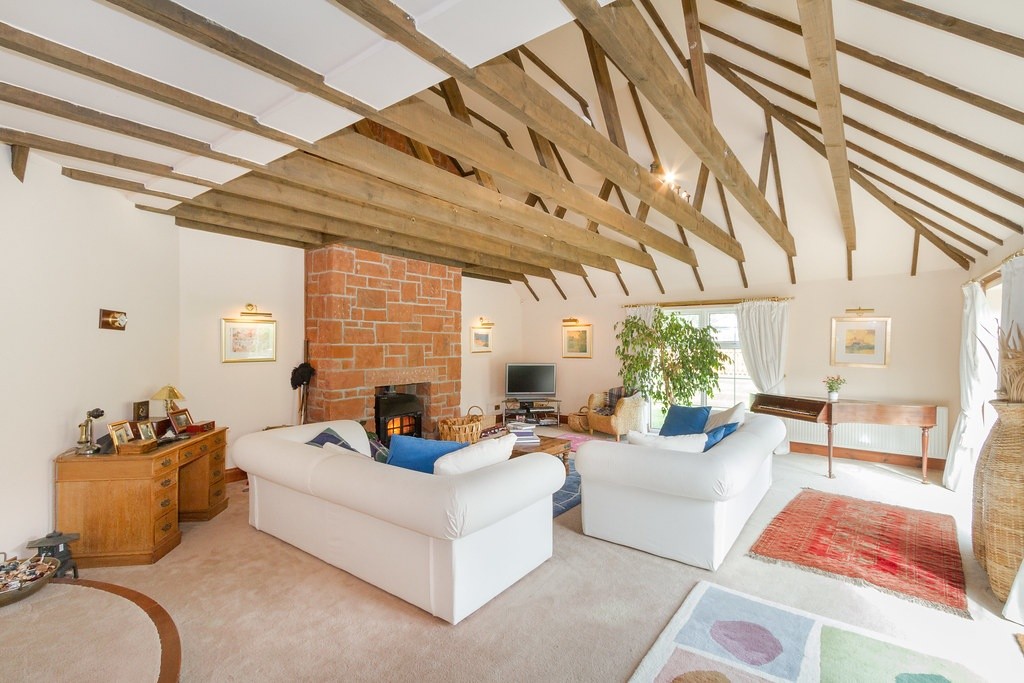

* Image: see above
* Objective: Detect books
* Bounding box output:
[506,421,541,443]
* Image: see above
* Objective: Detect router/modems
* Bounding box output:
[539,417,558,425]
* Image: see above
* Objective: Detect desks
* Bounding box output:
[51,423,233,570]
[501,396,563,428]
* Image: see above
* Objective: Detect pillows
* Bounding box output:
[627,399,747,456]
[303,425,470,475]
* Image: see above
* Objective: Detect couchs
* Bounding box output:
[229,416,569,629]
[586,386,644,443]
[579,407,788,574]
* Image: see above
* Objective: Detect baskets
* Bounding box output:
[438,406,484,445]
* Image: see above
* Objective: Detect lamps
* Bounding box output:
[661,175,691,203]
[149,385,186,420]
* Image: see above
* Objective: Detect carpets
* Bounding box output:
[623,578,988,683]
[741,485,975,624]
[555,432,610,453]
[552,457,581,520]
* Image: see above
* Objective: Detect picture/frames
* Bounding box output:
[219,317,277,362]
[107,401,195,454]
[829,313,892,369]
[469,325,493,353]
[561,323,594,360]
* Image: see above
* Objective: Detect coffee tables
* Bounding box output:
[510,434,572,477]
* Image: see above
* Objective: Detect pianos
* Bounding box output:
[750,392,940,485]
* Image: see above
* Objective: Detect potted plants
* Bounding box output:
[968,315,1024,606]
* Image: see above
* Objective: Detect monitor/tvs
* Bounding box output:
[505,363,557,394]
